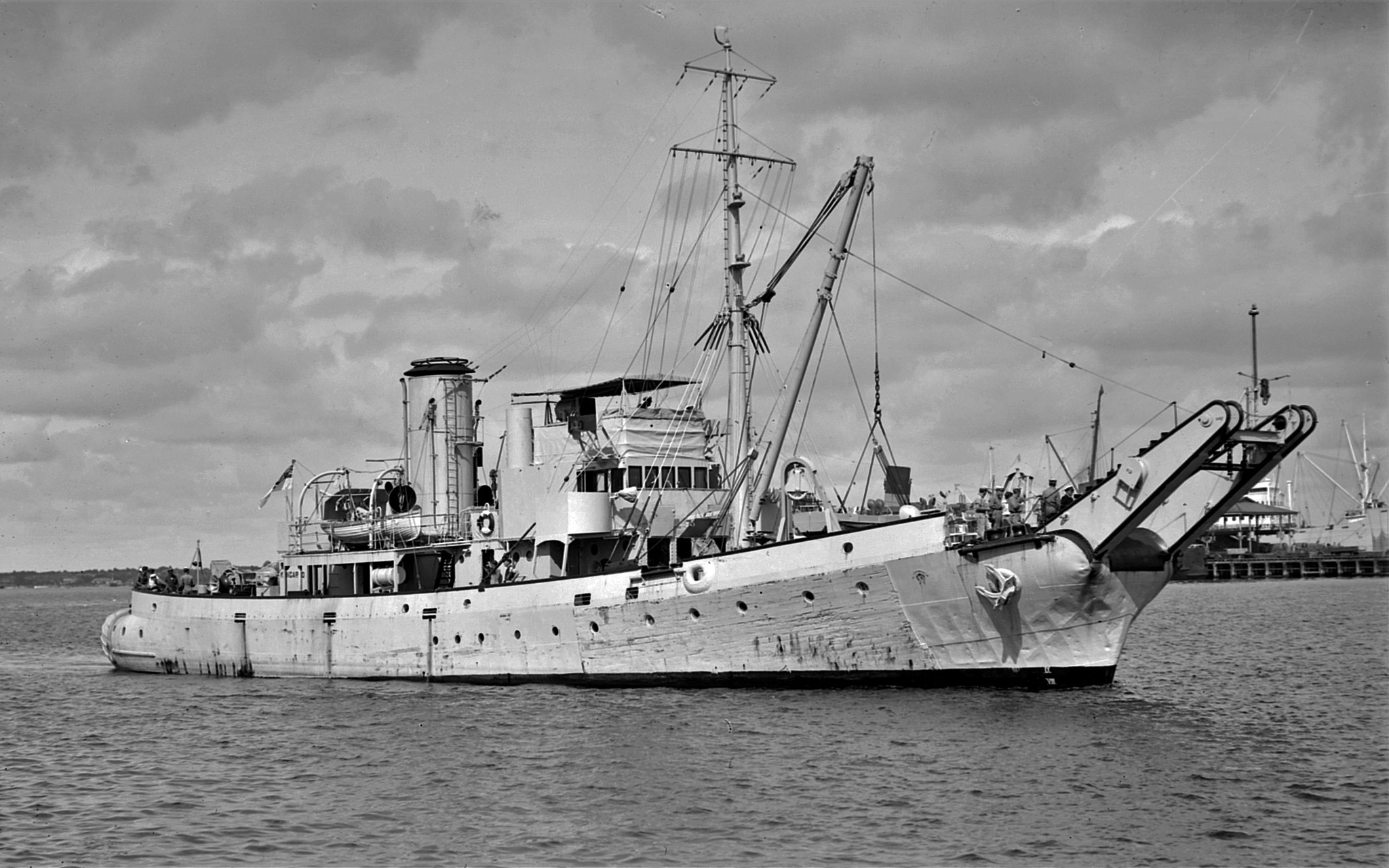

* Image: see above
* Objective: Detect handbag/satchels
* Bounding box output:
[498,573,503,583]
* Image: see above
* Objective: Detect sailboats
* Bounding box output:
[1247,379,1389,554]
[96,24,1320,695]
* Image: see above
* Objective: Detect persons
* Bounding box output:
[975,478,1075,542]
[136,566,195,595]
[488,557,519,585]
[637,396,652,408]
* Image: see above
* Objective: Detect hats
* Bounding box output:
[1064,486,1075,491]
[996,486,1004,490]
[506,559,512,563]
[1049,478,1056,484]
[1013,487,1021,491]
[979,486,989,491]
[183,567,189,571]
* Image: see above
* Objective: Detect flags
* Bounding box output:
[257,464,295,509]
